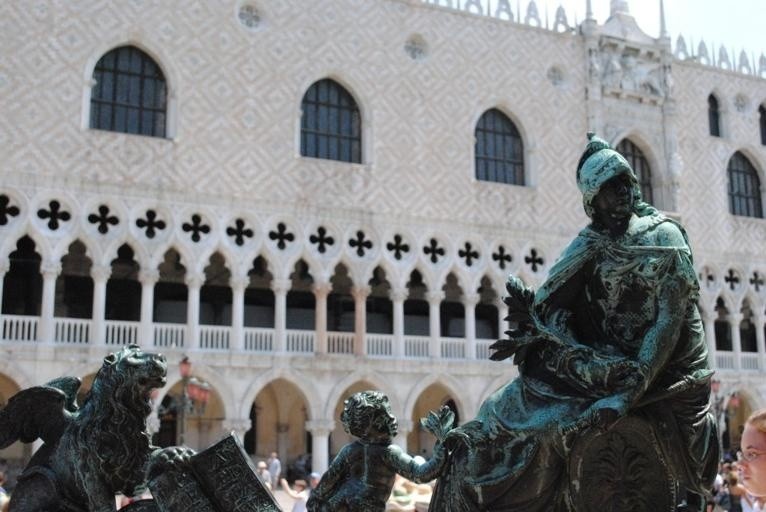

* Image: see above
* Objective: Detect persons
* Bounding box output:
[306,390,446,512]
[430,138,718,511]
[712,409,766,512]
[257,452,320,512]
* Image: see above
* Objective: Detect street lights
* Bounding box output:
[706,377,740,480]
[177,353,212,458]
